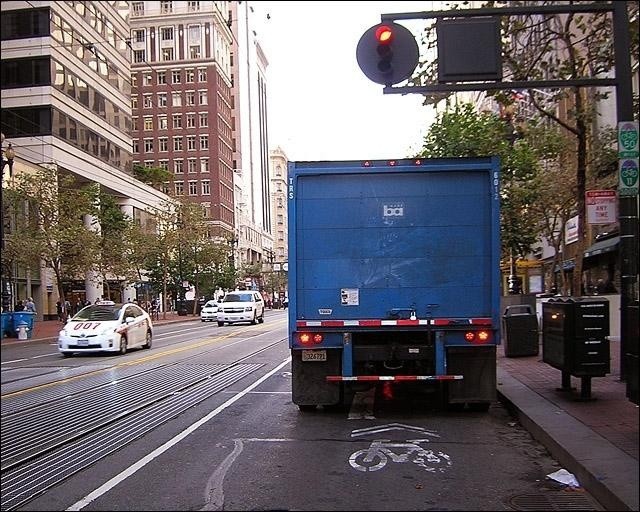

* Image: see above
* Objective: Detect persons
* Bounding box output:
[261,289,286,310]
[14,300,26,312]
[55,295,162,324]
[25,296,37,312]
[549,276,618,297]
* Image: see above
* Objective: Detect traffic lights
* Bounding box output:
[374,26,394,77]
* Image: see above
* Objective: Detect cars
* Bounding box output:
[200,300,219,323]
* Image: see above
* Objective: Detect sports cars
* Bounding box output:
[57,300,154,355]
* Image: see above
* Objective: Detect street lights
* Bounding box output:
[0,133,15,315]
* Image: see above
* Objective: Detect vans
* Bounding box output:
[217,290,265,324]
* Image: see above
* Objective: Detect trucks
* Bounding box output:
[286,155,503,412]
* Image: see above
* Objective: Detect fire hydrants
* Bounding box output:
[16,319,29,340]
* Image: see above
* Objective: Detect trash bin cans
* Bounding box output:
[502,304,540,358]
[0,311,37,340]
